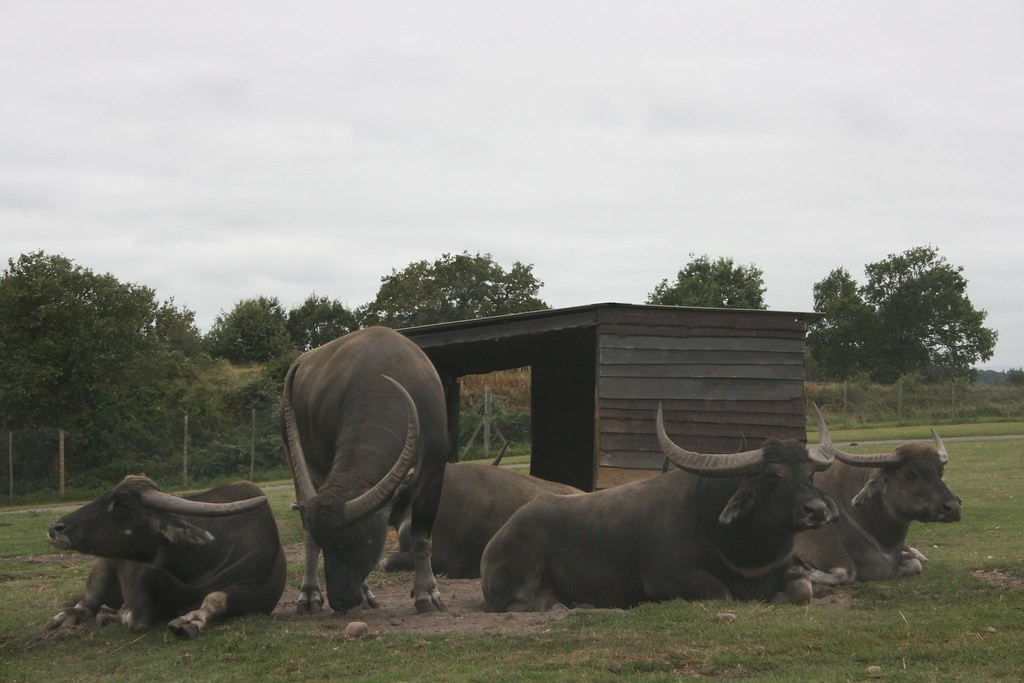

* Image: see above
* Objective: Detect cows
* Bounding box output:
[382,401,965,612]
[275,324,450,614]
[42,473,288,638]
